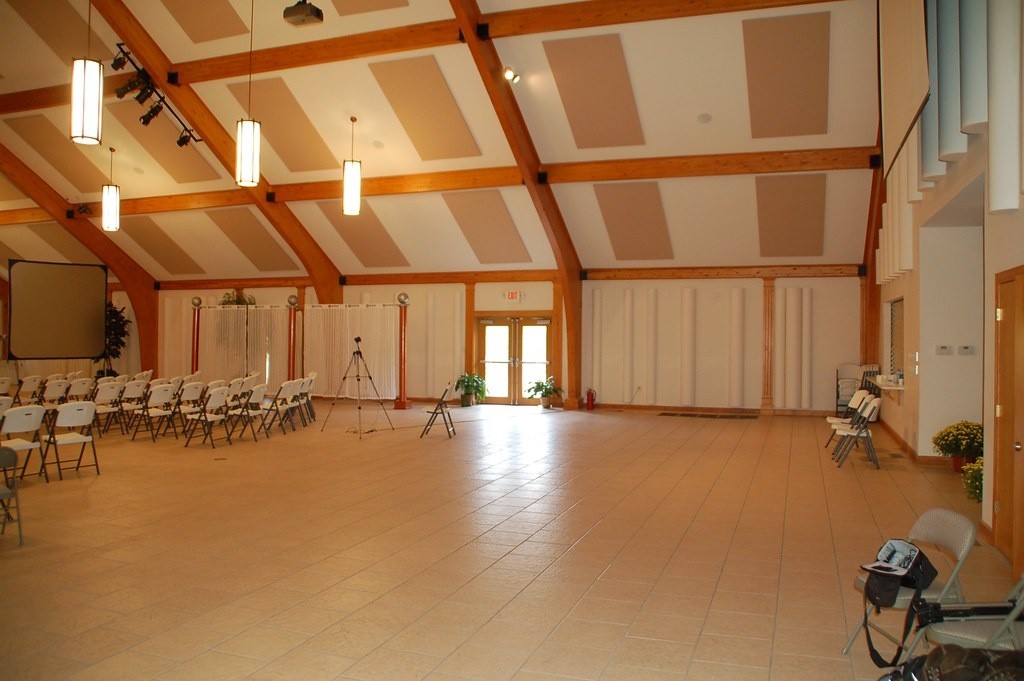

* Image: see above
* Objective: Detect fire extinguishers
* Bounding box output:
[587,388,596,411]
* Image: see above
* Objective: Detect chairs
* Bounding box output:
[823,362,883,469]
[841,506,977,667]
[419,381,456,439]
[899,575,1024,672]
[0,366,318,546]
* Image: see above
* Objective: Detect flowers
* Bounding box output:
[961,457,984,504]
[932,420,985,456]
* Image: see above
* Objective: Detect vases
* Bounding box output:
[953,453,976,474]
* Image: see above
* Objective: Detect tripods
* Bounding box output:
[320,337,394,439]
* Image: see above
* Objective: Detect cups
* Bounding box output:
[898,379,904,385]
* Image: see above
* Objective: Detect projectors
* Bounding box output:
[283,4,323,26]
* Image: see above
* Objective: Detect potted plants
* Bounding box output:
[526,375,565,408]
[454,371,488,406]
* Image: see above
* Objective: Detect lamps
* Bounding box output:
[101,148,121,232]
[69,0,104,146]
[342,116,362,217]
[234,0,262,188]
[111,42,205,146]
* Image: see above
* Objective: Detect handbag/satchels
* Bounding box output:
[859,538,938,669]
